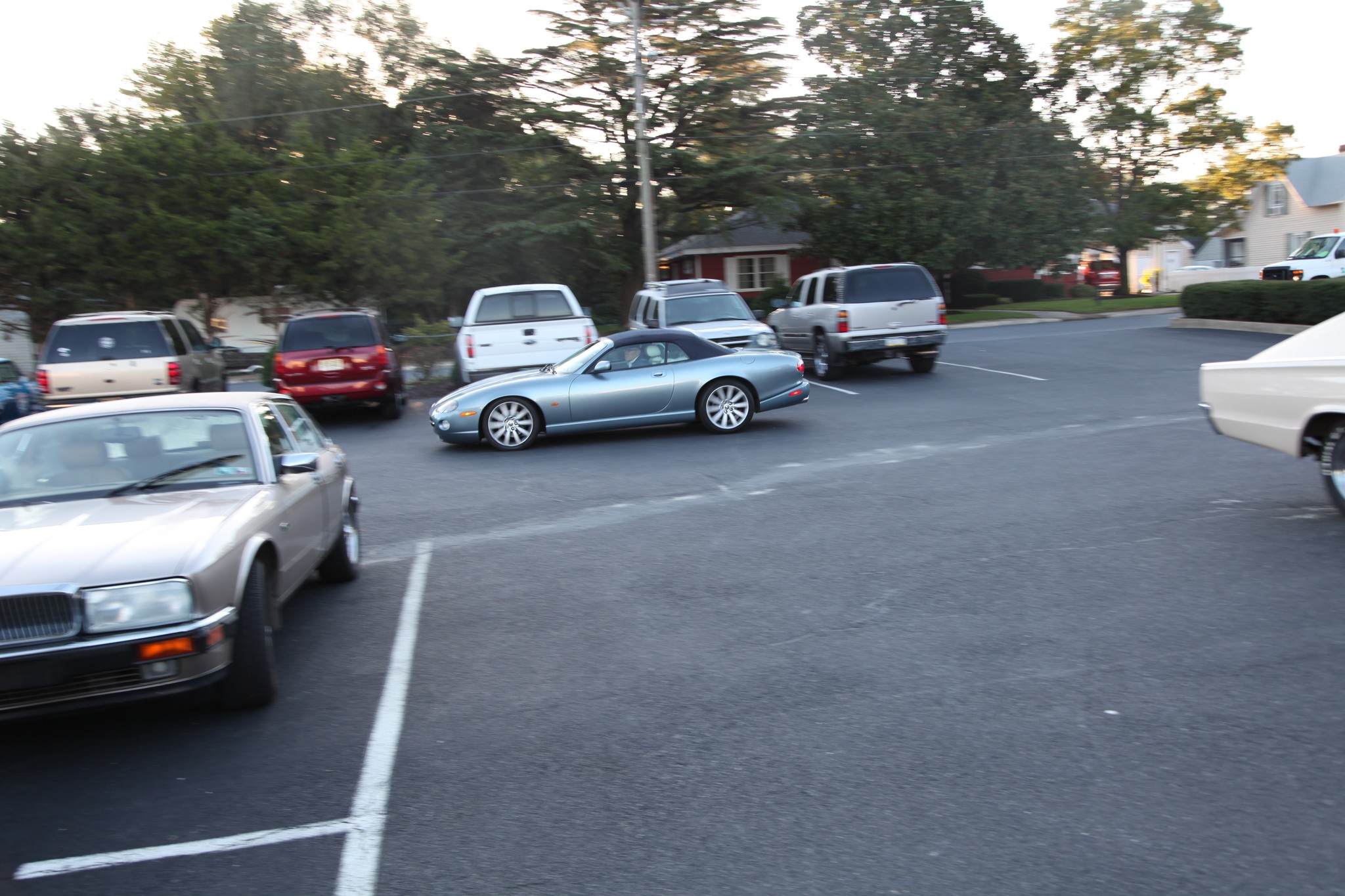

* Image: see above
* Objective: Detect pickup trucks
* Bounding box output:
[448,280,601,385]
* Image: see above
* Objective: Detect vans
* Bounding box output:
[1259,229,1345,280]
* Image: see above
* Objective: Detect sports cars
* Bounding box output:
[428,328,811,451]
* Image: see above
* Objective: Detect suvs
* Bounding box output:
[277,306,407,420]
[1077,259,1121,292]
[39,308,228,412]
[629,279,780,354]
[766,261,948,383]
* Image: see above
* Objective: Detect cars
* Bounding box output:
[0,357,29,424]
[0,391,360,728]
[1199,311,1345,513]
